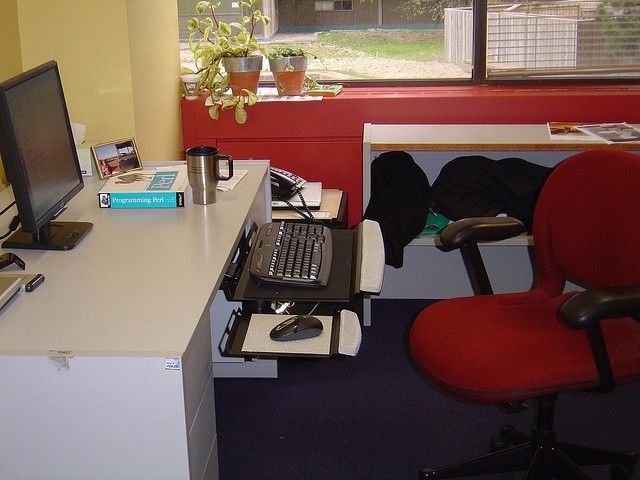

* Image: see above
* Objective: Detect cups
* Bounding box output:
[184,145,233,206]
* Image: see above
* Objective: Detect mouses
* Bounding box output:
[269,315,323,342]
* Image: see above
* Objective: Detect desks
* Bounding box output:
[1,158,386,480]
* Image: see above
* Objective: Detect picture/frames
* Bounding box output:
[91,136,143,180]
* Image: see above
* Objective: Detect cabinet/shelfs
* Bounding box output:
[362,122,640,327]
[272,188,349,229]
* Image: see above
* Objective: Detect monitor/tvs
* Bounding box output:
[0,60,94,251]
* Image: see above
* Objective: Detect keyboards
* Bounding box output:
[249,222,333,287]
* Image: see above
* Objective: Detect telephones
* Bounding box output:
[270,166,320,222]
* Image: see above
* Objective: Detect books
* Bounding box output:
[96,165,194,209]
[307,84,344,97]
[547,120,600,142]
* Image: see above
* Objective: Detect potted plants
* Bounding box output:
[267,45,329,97]
[180,1,271,124]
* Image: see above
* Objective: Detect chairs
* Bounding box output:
[405,148,640,480]
